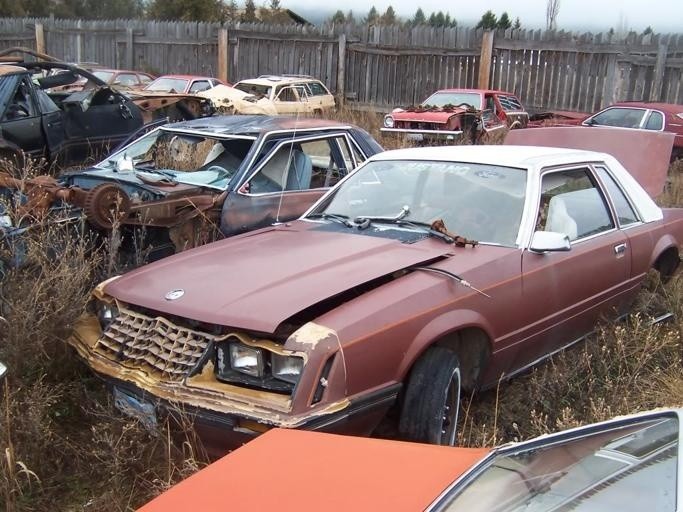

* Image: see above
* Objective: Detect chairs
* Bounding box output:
[521,193,578,244]
[257,150,313,194]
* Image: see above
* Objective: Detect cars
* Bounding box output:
[528,101,683,151]
[134,406,678,512]
[64,124,683,435]
[379,89,530,143]
[1,47,230,190]
[62,114,412,267]
[197,74,338,114]
